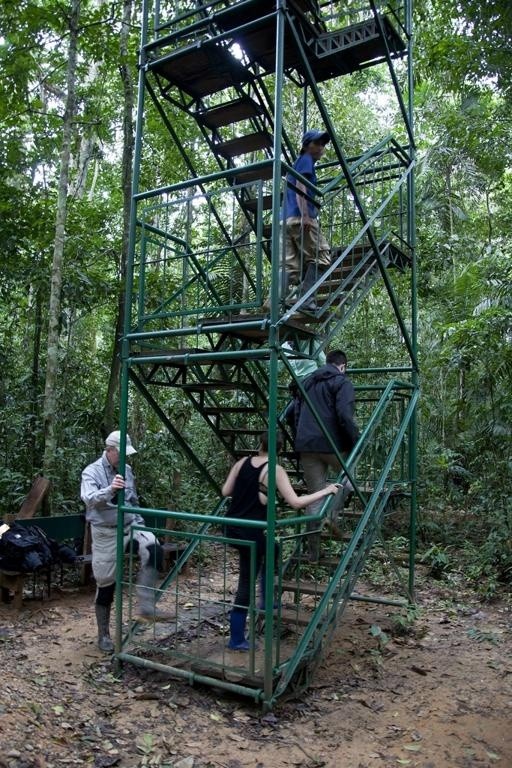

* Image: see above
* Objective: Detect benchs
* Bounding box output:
[0,503,190,608]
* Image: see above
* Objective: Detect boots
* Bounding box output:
[258,576,279,609]
[95,605,115,651]
[229,611,257,649]
[263,271,288,313]
[136,569,171,616]
[306,523,323,564]
[297,265,327,311]
[323,483,349,539]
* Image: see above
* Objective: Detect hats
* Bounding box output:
[303,131,330,144]
[105,430,137,456]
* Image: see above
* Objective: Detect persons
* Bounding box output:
[294,349,361,564]
[79,430,182,656]
[220,429,345,653]
[281,322,328,448]
[261,128,333,315]
